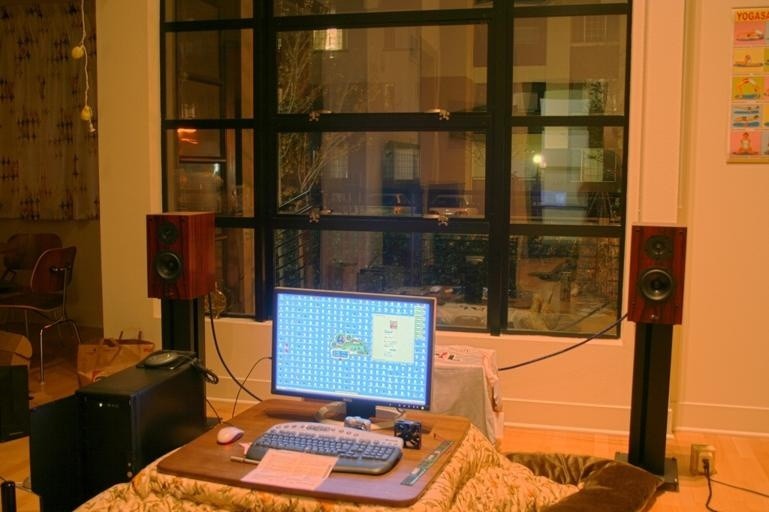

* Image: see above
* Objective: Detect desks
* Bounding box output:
[421,282,537,313]
[156,400,471,507]
[378,345,504,451]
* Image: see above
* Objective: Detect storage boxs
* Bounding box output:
[108,338,158,361]
[79,338,144,386]
[0,333,33,369]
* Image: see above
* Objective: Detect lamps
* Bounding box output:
[313,29,344,59]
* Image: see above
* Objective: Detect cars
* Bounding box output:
[361,191,417,218]
[427,193,482,221]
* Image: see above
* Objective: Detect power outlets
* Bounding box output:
[690,444,716,475]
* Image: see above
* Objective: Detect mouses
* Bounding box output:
[216,426,244,445]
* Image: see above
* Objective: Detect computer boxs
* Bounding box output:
[75,358,205,508]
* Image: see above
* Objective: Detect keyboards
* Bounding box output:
[246,421,405,475]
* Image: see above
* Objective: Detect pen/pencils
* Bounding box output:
[230,456,260,464]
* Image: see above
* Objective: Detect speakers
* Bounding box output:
[145,211,216,301]
[626,226,688,325]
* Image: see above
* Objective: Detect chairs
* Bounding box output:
[0,245,80,385]
[0,233,63,344]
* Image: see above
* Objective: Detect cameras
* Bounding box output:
[343,416,371,431]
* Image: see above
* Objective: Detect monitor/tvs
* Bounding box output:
[270,286,438,430]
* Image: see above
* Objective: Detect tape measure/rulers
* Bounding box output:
[399,439,455,485]
